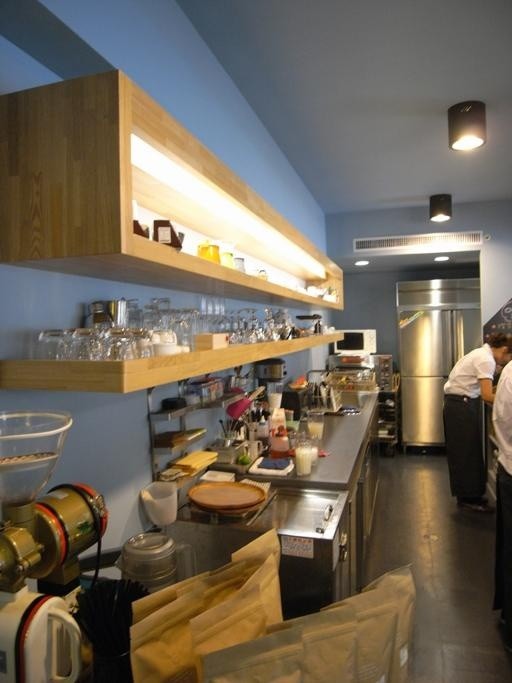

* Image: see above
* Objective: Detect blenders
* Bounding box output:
[253,359,289,412]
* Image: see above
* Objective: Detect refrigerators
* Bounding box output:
[396,278,483,447]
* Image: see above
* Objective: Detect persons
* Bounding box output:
[440,331,511,515]
[490,352,511,654]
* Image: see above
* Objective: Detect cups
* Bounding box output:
[197,242,221,265]
[309,411,325,440]
[247,441,262,458]
[40,328,152,363]
[294,430,319,477]
[140,479,178,528]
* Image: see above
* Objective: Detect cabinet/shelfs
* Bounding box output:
[0,67,345,396]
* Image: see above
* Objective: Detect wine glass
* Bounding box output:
[200,294,260,343]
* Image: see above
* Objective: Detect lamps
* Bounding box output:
[426,98,488,226]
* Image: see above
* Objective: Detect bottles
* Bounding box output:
[256,415,268,449]
[85,301,114,328]
[271,429,290,459]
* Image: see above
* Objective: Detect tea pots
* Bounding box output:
[115,532,198,590]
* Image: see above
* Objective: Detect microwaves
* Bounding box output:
[333,328,378,356]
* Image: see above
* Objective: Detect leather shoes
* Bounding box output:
[458,500,496,514]
